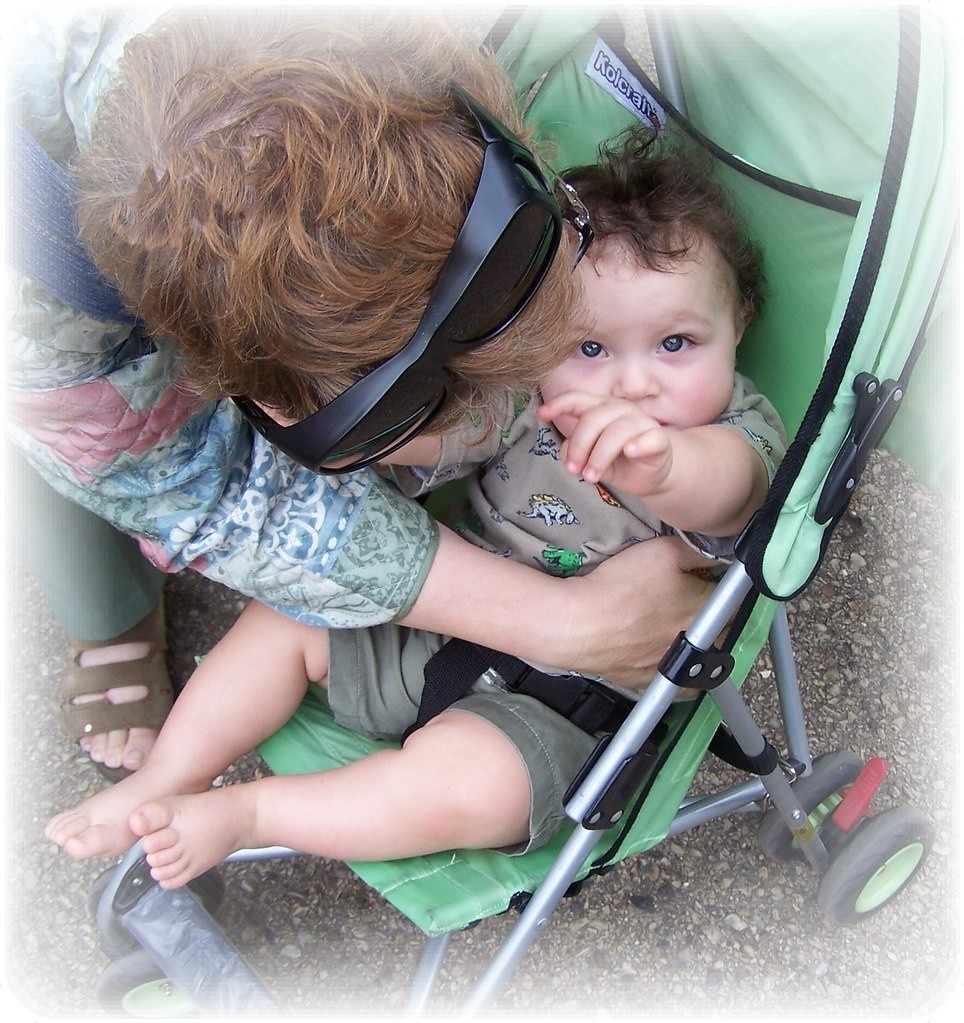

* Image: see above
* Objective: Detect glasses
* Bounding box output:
[222,73,565,478]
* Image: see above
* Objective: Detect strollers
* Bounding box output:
[90,0,960,1018]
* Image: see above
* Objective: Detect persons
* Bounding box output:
[17,7,755,784]
[46,118,792,898]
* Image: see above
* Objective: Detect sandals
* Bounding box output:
[60,581,178,788]
